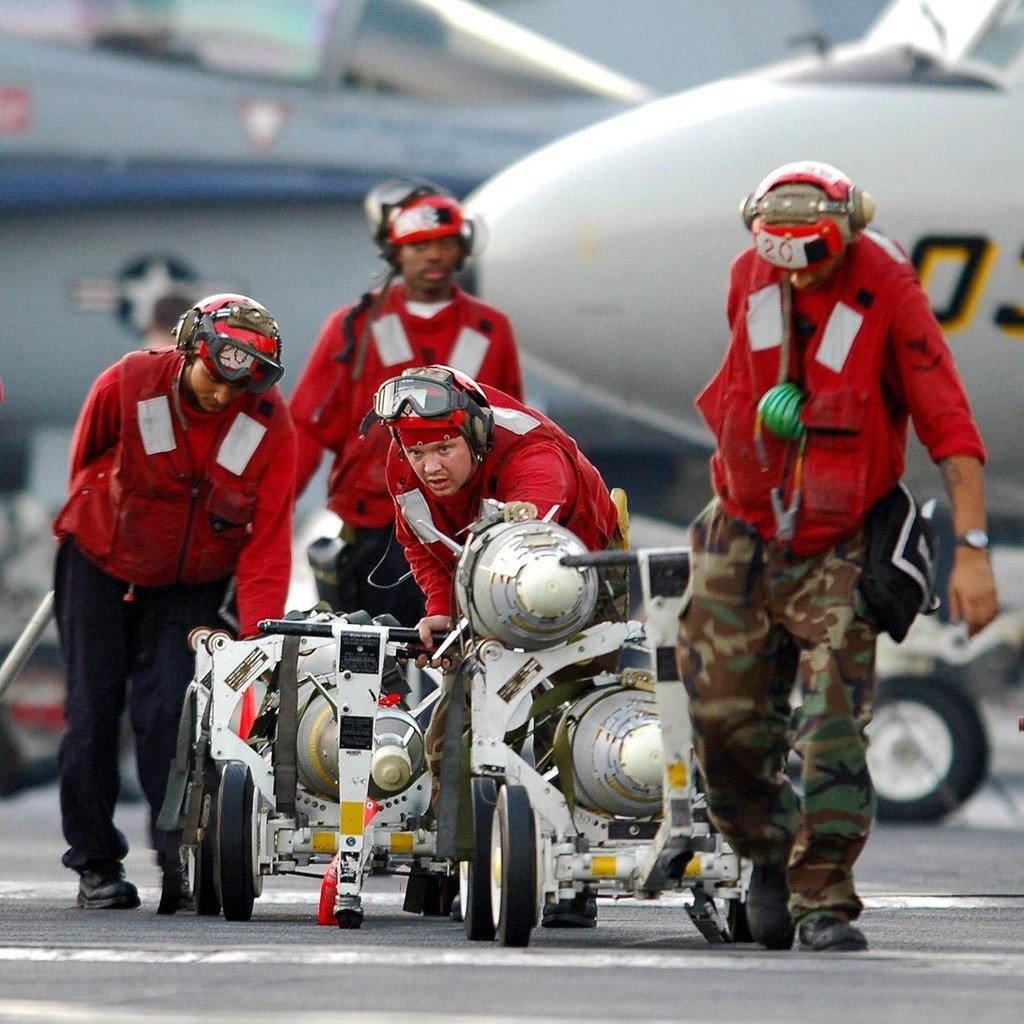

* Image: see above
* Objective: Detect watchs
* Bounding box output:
[951,528,990,550]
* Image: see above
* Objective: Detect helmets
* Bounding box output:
[738,160,877,273]
[170,293,282,393]
[381,189,465,245]
[380,363,495,464]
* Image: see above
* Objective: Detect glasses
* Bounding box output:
[362,177,421,236]
[372,375,470,418]
[208,336,284,394]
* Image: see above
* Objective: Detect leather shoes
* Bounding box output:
[76,860,142,909]
[156,864,197,915]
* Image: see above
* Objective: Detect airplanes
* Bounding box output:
[0,0,656,798]
[436,0,1024,822]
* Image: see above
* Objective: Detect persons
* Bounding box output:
[50,291,300,914]
[288,173,525,619]
[361,364,629,926]
[674,158,999,950]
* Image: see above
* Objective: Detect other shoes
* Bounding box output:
[744,864,796,951]
[799,916,869,952]
[541,891,598,928]
